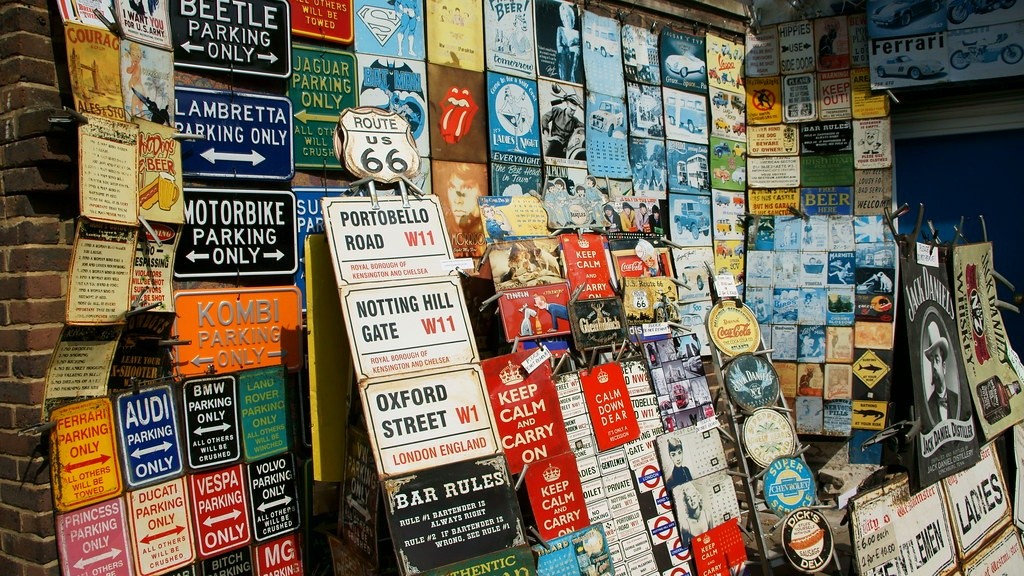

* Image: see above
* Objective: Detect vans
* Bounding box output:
[583,24,618,58]
[666,93,707,131]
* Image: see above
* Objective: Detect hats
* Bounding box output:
[924,322,949,360]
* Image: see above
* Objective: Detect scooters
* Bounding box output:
[949,33,1024,69]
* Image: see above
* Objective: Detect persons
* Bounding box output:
[922,321,958,424]
[818,29,838,61]
[754,300,767,319]
[508,243,536,280]
[605,202,661,235]
[541,98,584,159]
[555,2,582,84]
[683,485,713,548]
[447,163,488,257]
[665,437,692,490]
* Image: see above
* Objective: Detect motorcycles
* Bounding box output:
[946,0,1019,25]
[550,83,585,160]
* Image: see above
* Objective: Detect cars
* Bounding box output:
[871,0,943,29]
[666,51,706,77]
[876,55,944,78]
[590,101,626,137]
[674,200,710,239]
